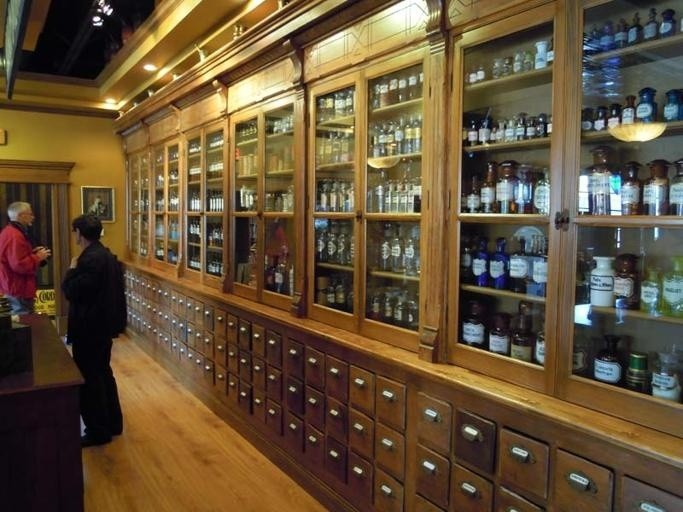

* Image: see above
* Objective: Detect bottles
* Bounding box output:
[235,115,258,290]
[131,141,178,263]
[459,297,545,364]
[313,87,354,312]
[263,103,295,297]
[204,129,224,276]
[574,326,682,400]
[461,111,553,145]
[464,38,553,86]
[460,160,550,215]
[579,87,681,129]
[460,234,548,297]
[575,247,682,319]
[185,136,201,270]
[364,63,423,331]
[578,144,682,216]
[581,5,683,53]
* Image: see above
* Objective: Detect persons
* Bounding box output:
[87,196,102,214]
[1,201,52,313]
[62,213,127,447]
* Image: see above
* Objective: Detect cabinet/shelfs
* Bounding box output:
[148,128,183,388]
[225,87,307,467]
[181,113,226,414]
[116,139,152,361]
[305,32,431,508]
[427,2,680,509]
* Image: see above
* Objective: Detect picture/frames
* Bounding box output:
[80,184,115,223]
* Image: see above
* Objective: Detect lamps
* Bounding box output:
[85,0,114,27]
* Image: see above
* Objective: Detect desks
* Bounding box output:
[0,313,87,512]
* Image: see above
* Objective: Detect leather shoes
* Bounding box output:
[81,434,100,447]
